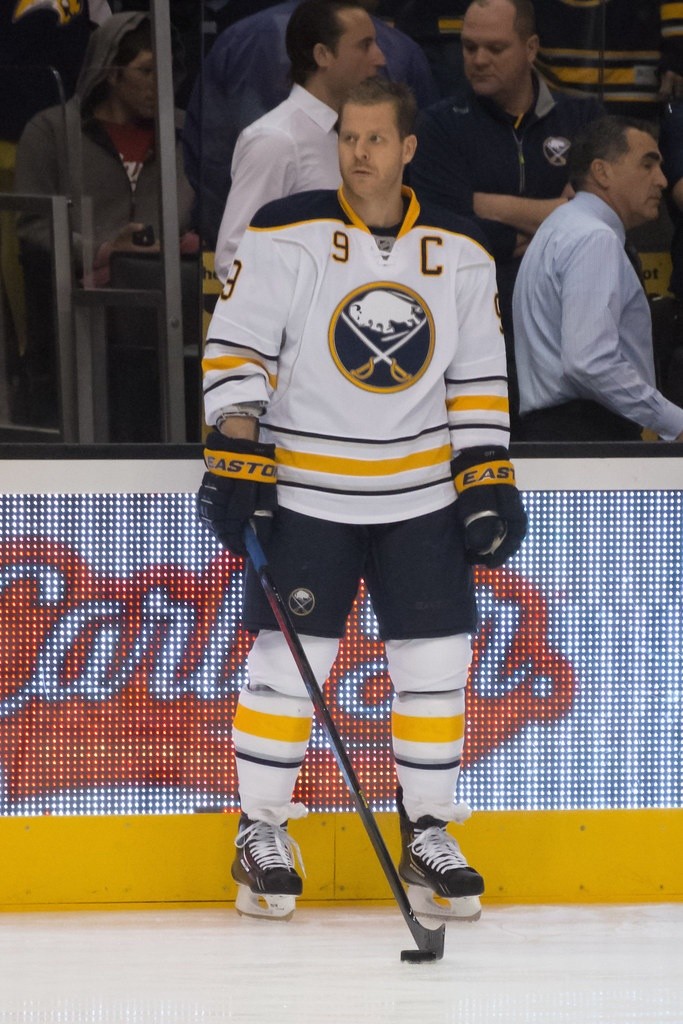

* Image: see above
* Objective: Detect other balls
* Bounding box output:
[400,948,438,963]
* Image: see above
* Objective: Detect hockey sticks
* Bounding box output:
[244,523,444,963]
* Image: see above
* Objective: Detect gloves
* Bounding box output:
[450,447,528,569]
[197,430,277,558]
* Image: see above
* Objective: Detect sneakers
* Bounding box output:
[229,814,308,918]
[396,786,485,917]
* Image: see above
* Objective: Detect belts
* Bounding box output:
[538,401,612,420]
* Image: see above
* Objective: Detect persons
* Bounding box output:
[185,0,439,252]
[511,117,683,441]
[413,0,646,319]
[198,79,529,920]
[0,0,204,442]
[214,0,388,284]
[656,97,683,299]
